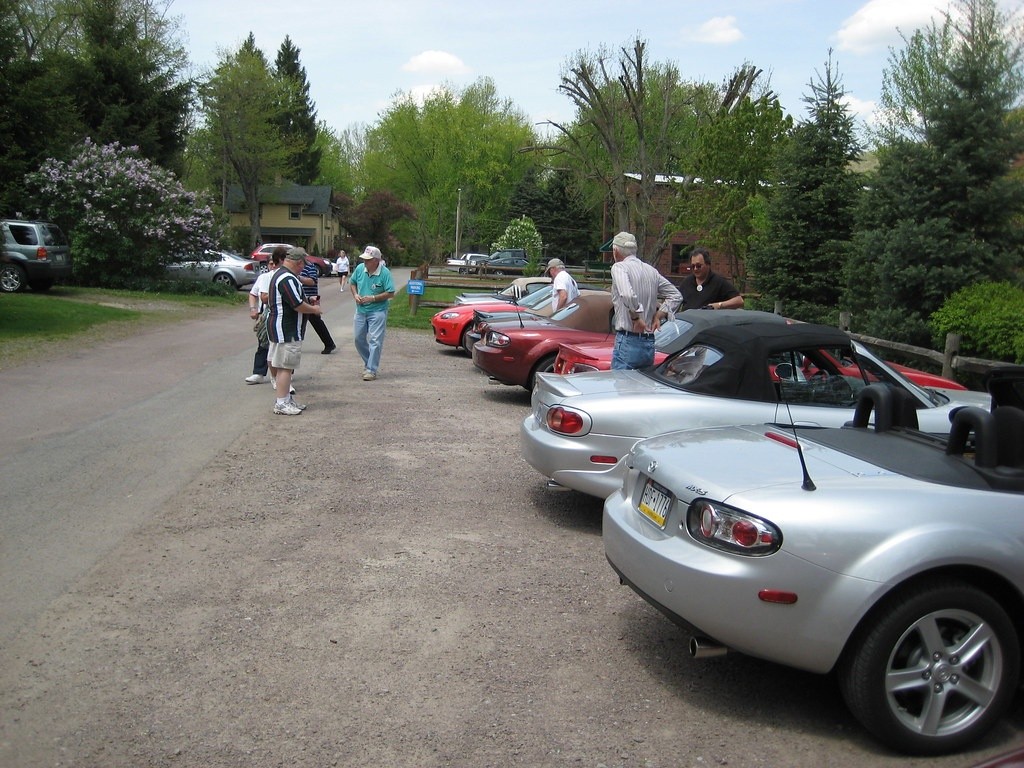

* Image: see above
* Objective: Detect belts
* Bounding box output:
[618,330,655,338]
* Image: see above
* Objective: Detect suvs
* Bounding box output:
[251,243,332,278]
[0,219,73,293]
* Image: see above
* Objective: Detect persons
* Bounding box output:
[544,258,580,314]
[245,243,336,416]
[349,246,394,379]
[679,248,745,318]
[332,249,350,293]
[609,231,684,372]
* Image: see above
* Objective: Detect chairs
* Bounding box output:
[881,380,918,430]
[990,405,1024,467]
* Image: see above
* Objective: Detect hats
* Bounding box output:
[544,258,565,273]
[286,247,310,260]
[610,232,637,247]
[359,246,381,259]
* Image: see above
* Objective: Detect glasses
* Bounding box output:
[268,263,277,266]
[691,263,707,269]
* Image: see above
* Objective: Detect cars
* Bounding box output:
[453,276,562,306]
[483,258,548,281]
[520,324,993,498]
[603,365,1024,757]
[473,291,615,391]
[465,291,611,354]
[553,310,968,391]
[432,283,607,354]
[158,249,260,290]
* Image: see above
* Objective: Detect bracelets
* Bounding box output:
[307,296,311,303]
[630,316,640,321]
[372,295,376,302]
[250,306,258,308]
[718,302,722,309]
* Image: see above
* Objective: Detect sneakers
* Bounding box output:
[289,399,306,410]
[321,342,336,354]
[363,367,376,380]
[273,402,302,415]
[245,374,264,384]
[271,378,277,389]
[289,384,296,393]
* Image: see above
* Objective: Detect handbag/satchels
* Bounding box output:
[253,291,271,350]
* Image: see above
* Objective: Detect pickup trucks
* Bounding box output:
[480,248,554,265]
[445,254,489,275]
